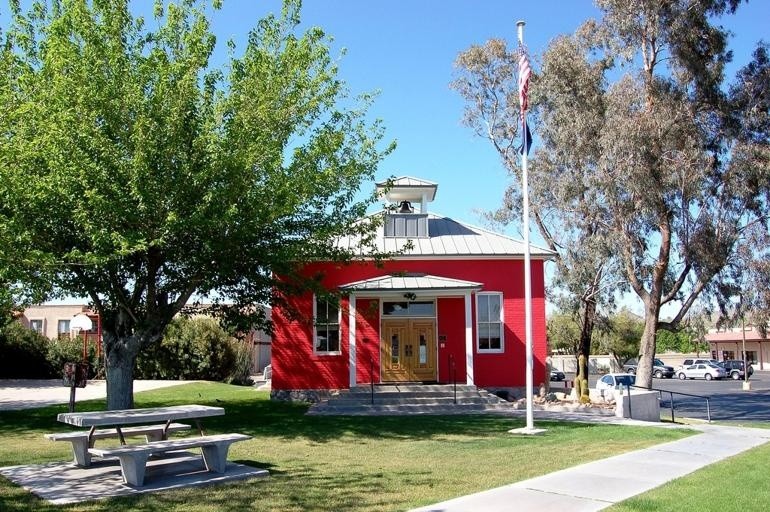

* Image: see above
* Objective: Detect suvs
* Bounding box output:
[622,356,674,379]
[716,360,753,380]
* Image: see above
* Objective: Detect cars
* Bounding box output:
[596,372,636,393]
[676,363,726,381]
[547,368,565,381]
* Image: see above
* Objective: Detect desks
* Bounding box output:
[58,404,226,446]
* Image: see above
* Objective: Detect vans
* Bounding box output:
[683,358,723,368]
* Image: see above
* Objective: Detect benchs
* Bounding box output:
[45,422,191,466]
[87,431,253,487]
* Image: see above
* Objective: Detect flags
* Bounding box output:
[515,38,533,157]
